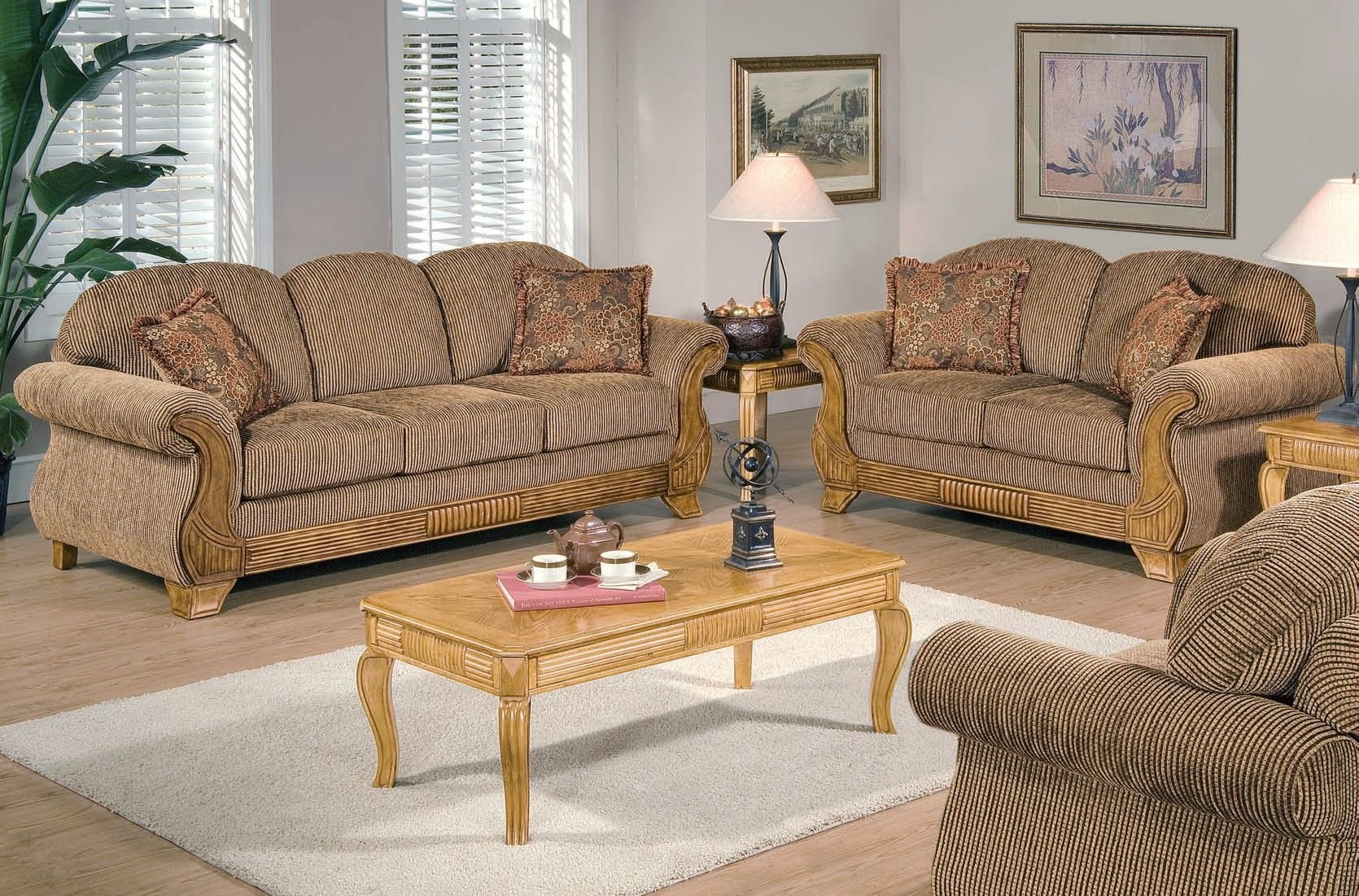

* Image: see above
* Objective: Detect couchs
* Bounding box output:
[907,482,1359,896]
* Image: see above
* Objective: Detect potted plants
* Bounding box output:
[0,0,241,538]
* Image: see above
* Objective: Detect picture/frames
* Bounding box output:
[1013,22,1238,241]
[732,54,880,206]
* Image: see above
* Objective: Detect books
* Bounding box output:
[495,572,666,612]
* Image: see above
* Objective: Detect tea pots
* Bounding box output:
[547,509,624,574]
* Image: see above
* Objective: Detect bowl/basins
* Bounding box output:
[702,299,786,352]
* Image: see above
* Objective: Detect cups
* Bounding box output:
[600,550,639,576]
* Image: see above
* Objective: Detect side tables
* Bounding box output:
[1254,412,1359,510]
[703,346,826,502]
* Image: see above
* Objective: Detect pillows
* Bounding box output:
[1108,275,1222,408]
[884,256,1030,377]
[508,264,653,379]
[130,287,289,433]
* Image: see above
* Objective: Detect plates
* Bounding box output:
[524,553,567,581]
[515,569,578,591]
[589,565,651,578]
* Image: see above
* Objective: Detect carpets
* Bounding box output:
[0,581,1148,896]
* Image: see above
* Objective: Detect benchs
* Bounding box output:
[13,241,731,625]
[797,238,1358,587]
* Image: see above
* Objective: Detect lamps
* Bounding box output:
[1262,174,1359,428]
[710,151,844,345]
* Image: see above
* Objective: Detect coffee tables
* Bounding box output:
[357,518,912,847]
